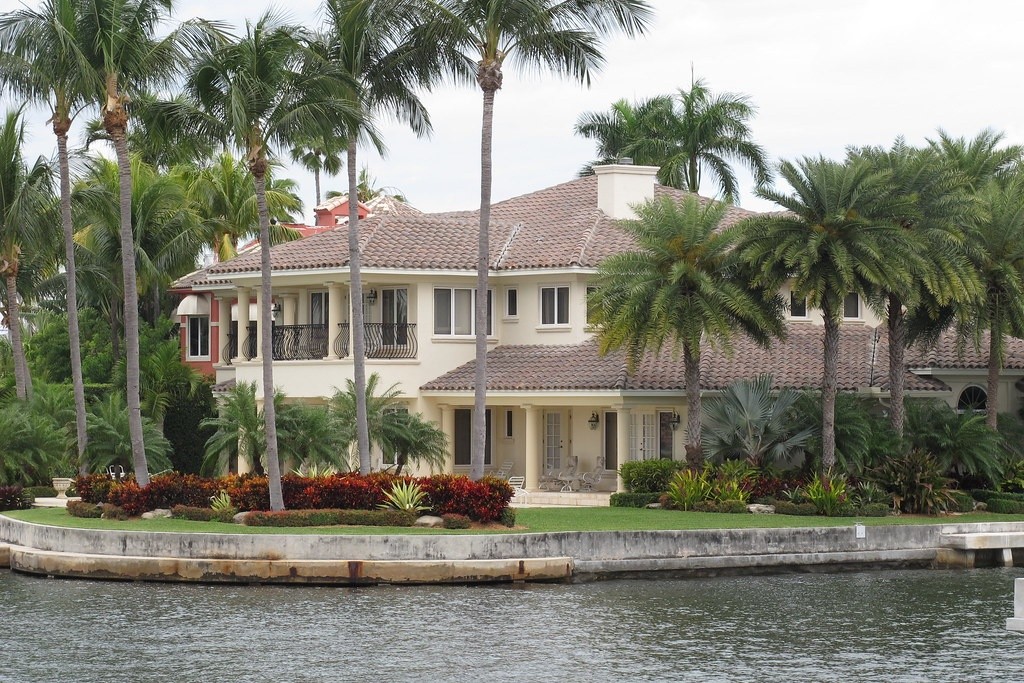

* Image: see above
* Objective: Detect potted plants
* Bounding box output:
[48,461,75,498]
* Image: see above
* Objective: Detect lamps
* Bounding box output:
[588,411,599,430]
[669,409,681,432]
[366,288,378,306]
[271,304,281,318]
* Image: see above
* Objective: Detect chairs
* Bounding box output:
[489,462,513,479]
[539,455,605,492]
[507,476,527,504]
